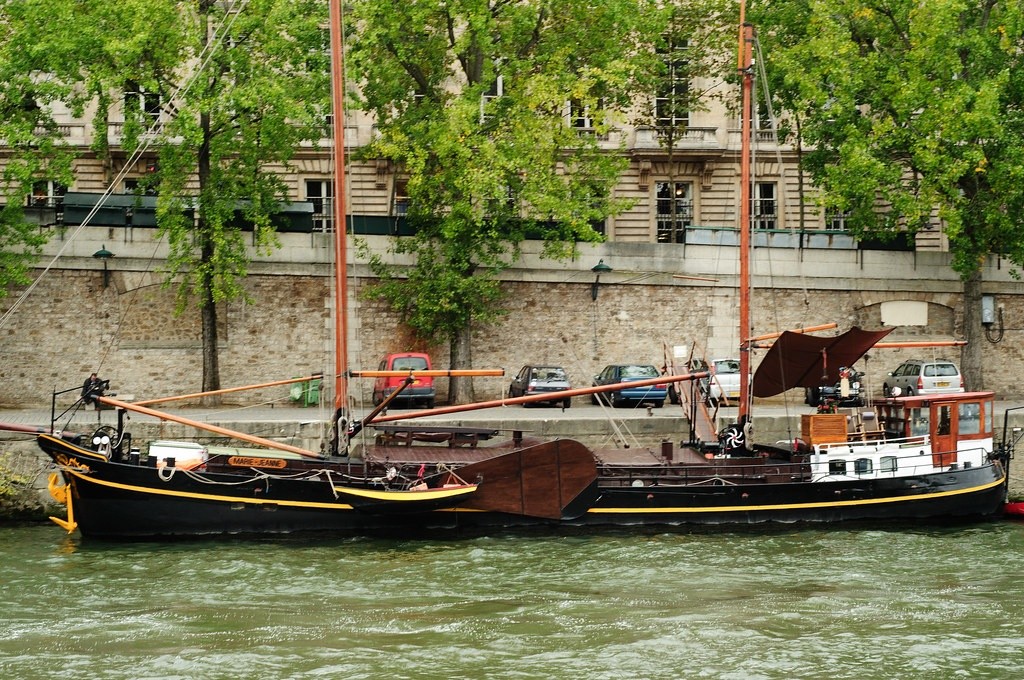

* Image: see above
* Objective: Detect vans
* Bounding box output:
[370,351,435,410]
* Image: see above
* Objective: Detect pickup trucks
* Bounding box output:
[668,357,753,402]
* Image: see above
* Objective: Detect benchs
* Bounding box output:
[77,394,136,411]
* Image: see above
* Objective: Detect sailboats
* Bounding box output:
[35,0,1024,556]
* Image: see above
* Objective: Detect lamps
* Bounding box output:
[92,243,116,289]
[590,258,613,302]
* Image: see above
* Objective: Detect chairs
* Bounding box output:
[837,406,888,445]
[532,373,538,379]
[730,363,738,370]
[547,373,558,379]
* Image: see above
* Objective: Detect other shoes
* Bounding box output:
[96,407,101,411]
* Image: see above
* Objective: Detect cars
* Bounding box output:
[590,364,668,409]
[509,364,571,408]
[806,367,865,407]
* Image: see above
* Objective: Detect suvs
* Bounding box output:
[884,358,966,394]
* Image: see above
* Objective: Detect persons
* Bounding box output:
[82,373,103,411]
